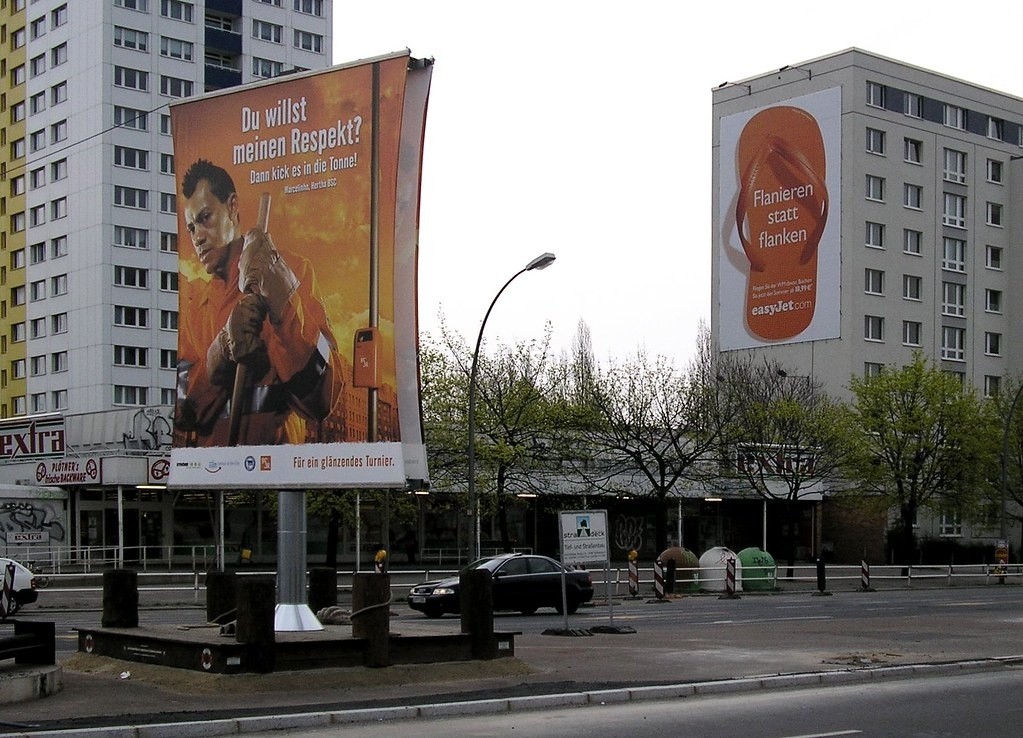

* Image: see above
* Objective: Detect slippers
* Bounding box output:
[736,106,829,340]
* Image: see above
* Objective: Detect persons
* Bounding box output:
[176,158,343,446]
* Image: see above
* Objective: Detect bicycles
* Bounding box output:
[7,554,49,588]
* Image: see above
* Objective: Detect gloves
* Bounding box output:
[207,293,271,386]
[237,227,301,324]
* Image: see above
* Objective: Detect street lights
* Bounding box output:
[464,252,558,559]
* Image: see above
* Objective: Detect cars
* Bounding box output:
[407,553,594,614]
[0,559,38,614]
